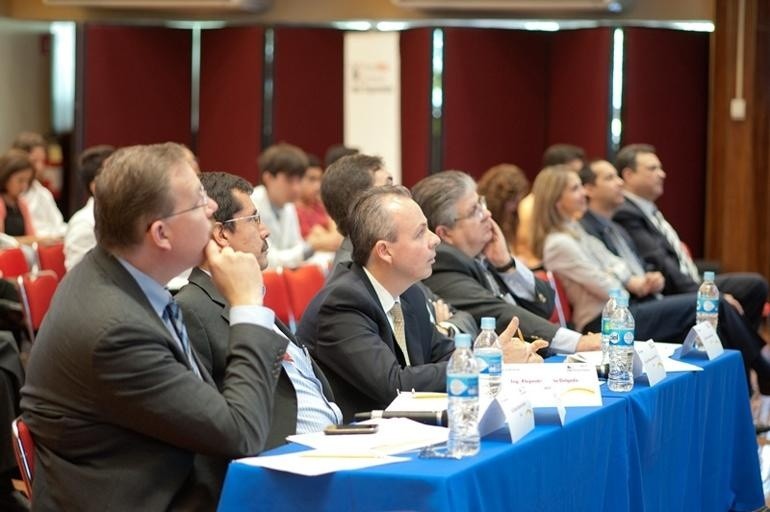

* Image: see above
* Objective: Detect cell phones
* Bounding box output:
[324,424,379,434]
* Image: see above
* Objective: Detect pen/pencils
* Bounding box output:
[516,327,526,341]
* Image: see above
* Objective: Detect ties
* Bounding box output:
[166,295,190,356]
[607,225,642,277]
[389,303,405,348]
[653,209,700,285]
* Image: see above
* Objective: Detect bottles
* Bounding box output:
[473,317,503,396]
[695,271,720,341]
[445,332,482,457]
[600,289,636,392]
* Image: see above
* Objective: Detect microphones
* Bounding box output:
[595,364,609,379]
[355,410,448,428]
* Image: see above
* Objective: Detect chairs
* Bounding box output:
[11,419,43,502]
[260,264,326,329]
[1,239,67,333]
[534,270,575,325]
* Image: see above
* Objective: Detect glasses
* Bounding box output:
[455,194,487,221]
[146,185,208,232]
[224,212,261,225]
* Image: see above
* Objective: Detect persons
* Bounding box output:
[476,143,770,397]
[20,142,289,512]
[169,172,344,450]
[0,138,115,272]
[250,141,601,425]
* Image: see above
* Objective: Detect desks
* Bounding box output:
[234,341,740,511]
